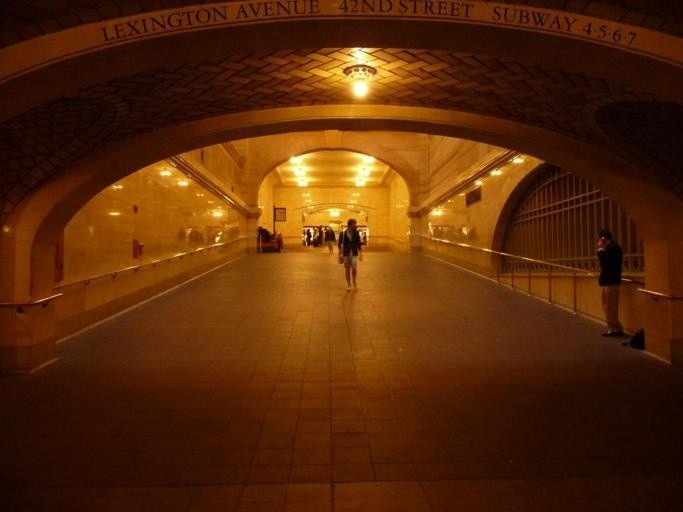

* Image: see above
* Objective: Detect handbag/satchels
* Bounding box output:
[338,256,343,264]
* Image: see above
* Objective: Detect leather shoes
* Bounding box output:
[601,330,622,337]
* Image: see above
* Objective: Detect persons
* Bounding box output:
[338,219,363,291]
[307,226,336,255]
[596,229,623,337]
